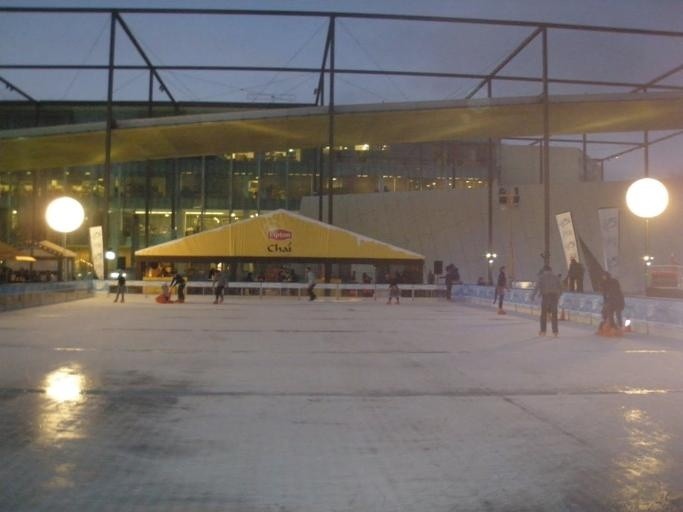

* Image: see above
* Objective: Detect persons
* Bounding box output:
[668,254,680,266]
[277,270,286,295]
[572,263,584,293]
[287,269,296,295]
[0,266,61,283]
[403,270,413,298]
[158,265,172,276]
[599,270,626,328]
[256,272,265,296]
[426,269,434,297]
[112,270,126,303]
[564,259,576,292]
[444,274,452,299]
[530,264,562,338]
[476,276,485,286]
[495,265,509,315]
[212,269,226,304]
[385,272,401,305]
[168,272,186,304]
[243,272,252,296]
[303,266,317,301]
[362,272,371,297]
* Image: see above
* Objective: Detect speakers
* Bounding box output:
[116,257,126,269]
[433,261,443,274]
